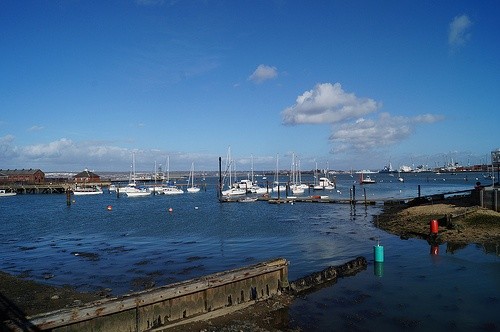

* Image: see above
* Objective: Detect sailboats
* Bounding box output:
[107,155,201,197]
[214,151,500,204]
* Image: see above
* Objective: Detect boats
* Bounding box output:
[77,186,104,195]
[0,190,17,196]
